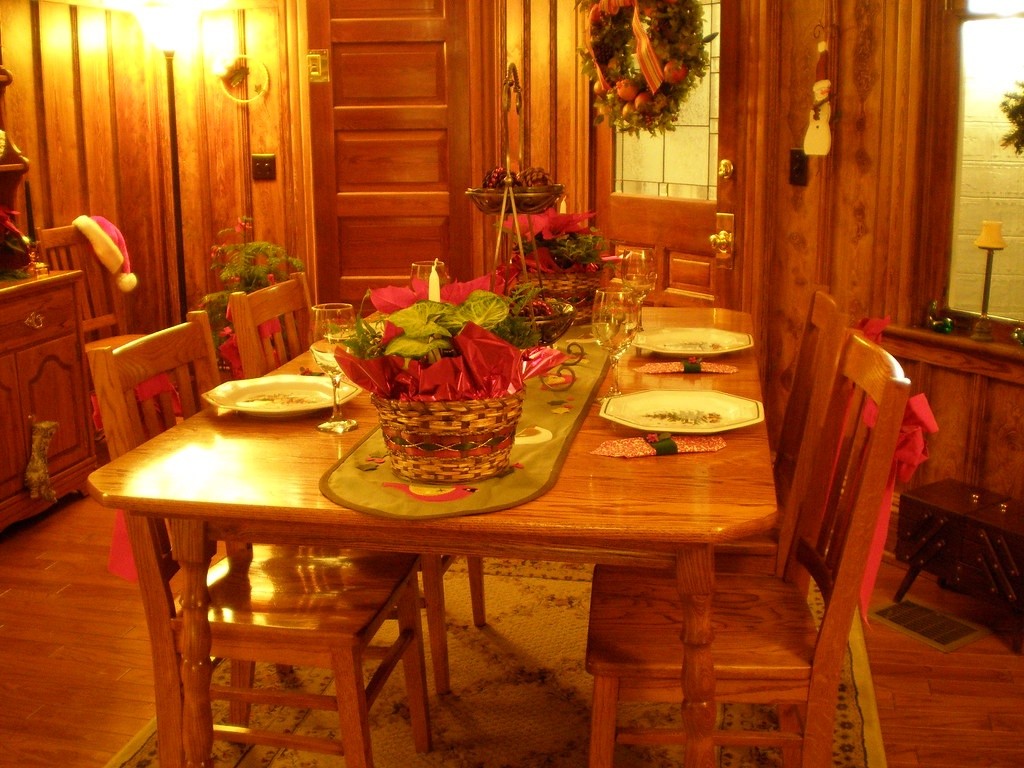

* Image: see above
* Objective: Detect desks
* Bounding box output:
[88,306,782,768]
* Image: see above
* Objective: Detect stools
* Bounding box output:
[893,480,1024,602]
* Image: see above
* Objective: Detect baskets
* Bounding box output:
[371,384,529,485]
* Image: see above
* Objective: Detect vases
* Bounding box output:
[216,359,234,385]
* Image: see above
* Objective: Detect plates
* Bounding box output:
[201,374,363,418]
[599,389,764,433]
[632,327,754,359]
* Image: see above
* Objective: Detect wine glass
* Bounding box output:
[593,286,635,404]
[621,247,657,331]
[310,303,358,433]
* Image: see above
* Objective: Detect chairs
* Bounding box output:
[239,263,491,687]
[83,311,433,768]
[608,288,858,568]
[583,326,912,768]
[37,226,149,353]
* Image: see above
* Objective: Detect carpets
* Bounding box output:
[102,561,889,768]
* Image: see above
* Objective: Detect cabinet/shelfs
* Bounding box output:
[0,157,99,531]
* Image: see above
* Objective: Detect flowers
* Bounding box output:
[334,274,562,401]
[199,218,305,351]
[504,209,612,274]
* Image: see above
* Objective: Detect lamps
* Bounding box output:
[973,221,1004,341]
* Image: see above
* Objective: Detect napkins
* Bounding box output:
[593,435,726,457]
[661,361,738,375]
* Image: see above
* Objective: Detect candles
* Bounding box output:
[427,257,441,304]
[561,194,568,215]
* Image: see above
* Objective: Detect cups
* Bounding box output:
[410,261,445,287]
